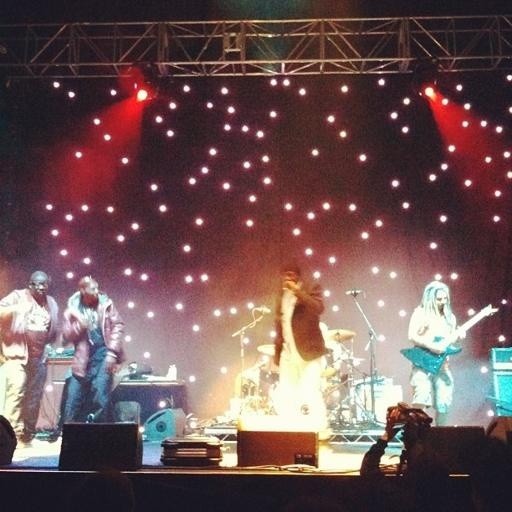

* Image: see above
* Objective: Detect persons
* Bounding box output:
[69,466,136,512]
[360,402,471,512]
[0,270,61,444]
[272,258,333,443]
[465,435,512,512]
[58,276,125,444]
[402,281,466,425]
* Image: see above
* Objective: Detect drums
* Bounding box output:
[323,382,348,407]
[257,365,279,396]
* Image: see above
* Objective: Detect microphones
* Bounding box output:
[255,307,271,314]
[346,289,360,298]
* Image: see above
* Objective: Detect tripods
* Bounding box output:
[322,299,389,428]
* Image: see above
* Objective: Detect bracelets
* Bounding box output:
[106,352,118,359]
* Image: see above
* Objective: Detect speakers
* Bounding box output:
[237,430,319,469]
[58,422,142,471]
[430,426,484,475]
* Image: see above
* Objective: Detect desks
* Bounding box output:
[34,357,74,431]
[48,381,187,429]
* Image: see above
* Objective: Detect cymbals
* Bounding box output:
[257,344,275,358]
[326,328,355,342]
[321,367,335,379]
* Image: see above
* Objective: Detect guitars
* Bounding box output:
[400,304,498,375]
